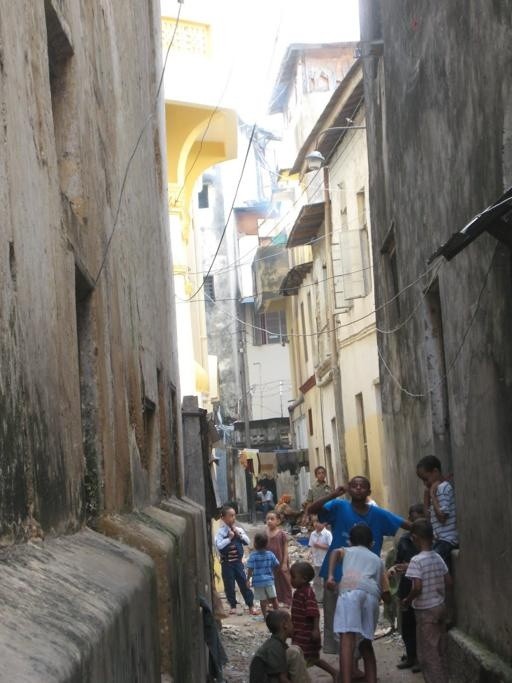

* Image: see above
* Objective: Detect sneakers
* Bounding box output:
[412,666,420,673]
[229,608,235,613]
[396,656,419,668]
[249,607,260,615]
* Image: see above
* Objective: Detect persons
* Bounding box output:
[215,454,459,683]
